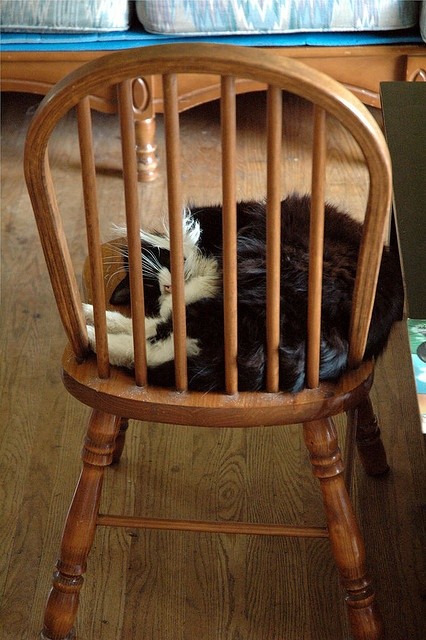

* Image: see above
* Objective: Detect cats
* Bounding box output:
[81,190,401,393]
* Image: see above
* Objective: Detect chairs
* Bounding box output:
[21,44,394,636]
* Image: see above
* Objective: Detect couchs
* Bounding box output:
[1,0,426,181]
